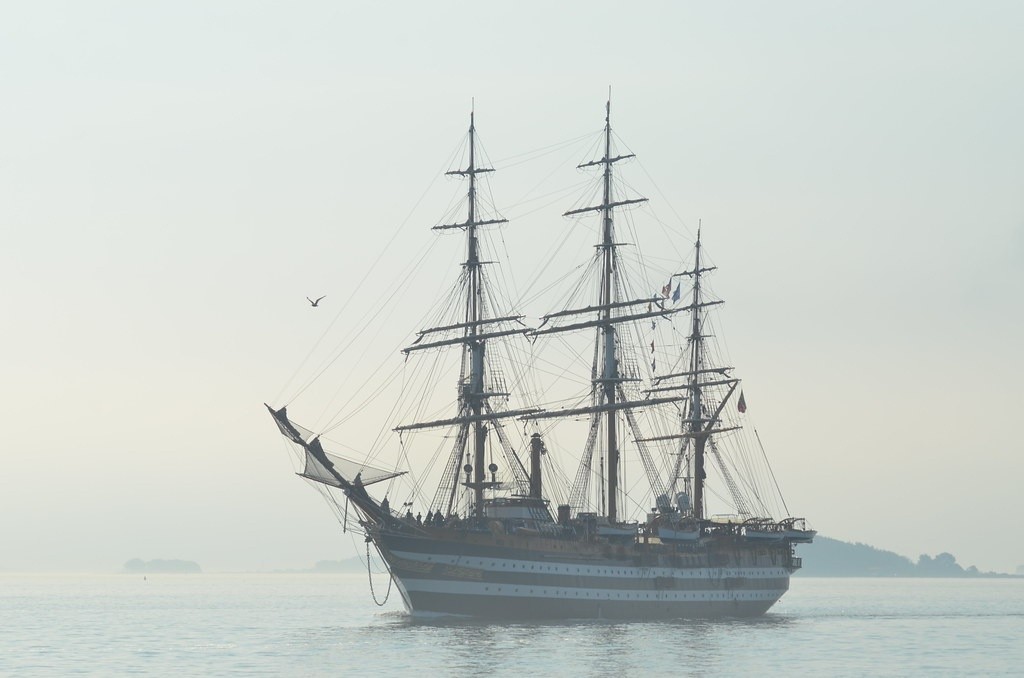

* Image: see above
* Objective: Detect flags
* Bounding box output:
[651,357,655,372]
[651,321,655,330]
[662,280,671,296]
[651,341,654,353]
[672,283,680,304]
[738,389,746,413]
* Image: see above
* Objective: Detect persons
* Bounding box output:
[427,510,458,521]
[727,520,734,535]
[406,509,410,517]
[416,512,421,521]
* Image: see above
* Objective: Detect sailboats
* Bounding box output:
[261,83,816,618]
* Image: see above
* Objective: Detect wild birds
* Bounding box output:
[307,296,326,307]
